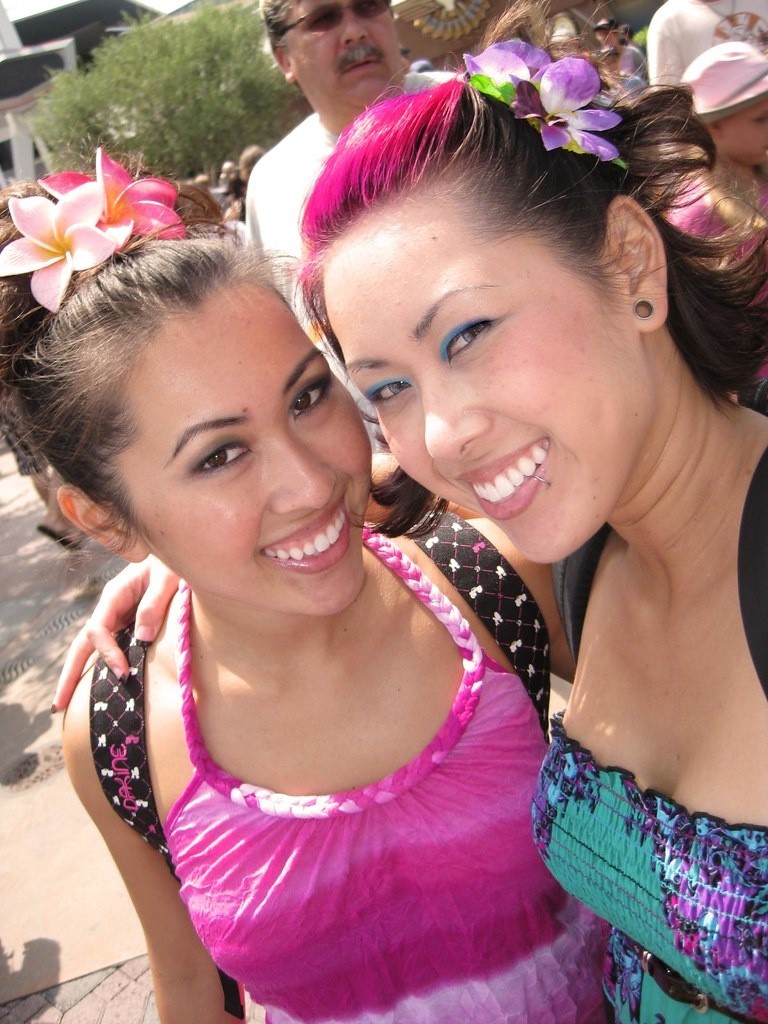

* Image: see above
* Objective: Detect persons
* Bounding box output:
[1,0,767,1023]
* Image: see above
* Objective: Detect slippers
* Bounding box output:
[37,525,83,551]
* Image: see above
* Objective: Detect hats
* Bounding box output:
[619,24,634,36]
[591,17,619,32]
[681,40,768,126]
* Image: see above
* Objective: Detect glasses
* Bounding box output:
[275,0,392,36]
